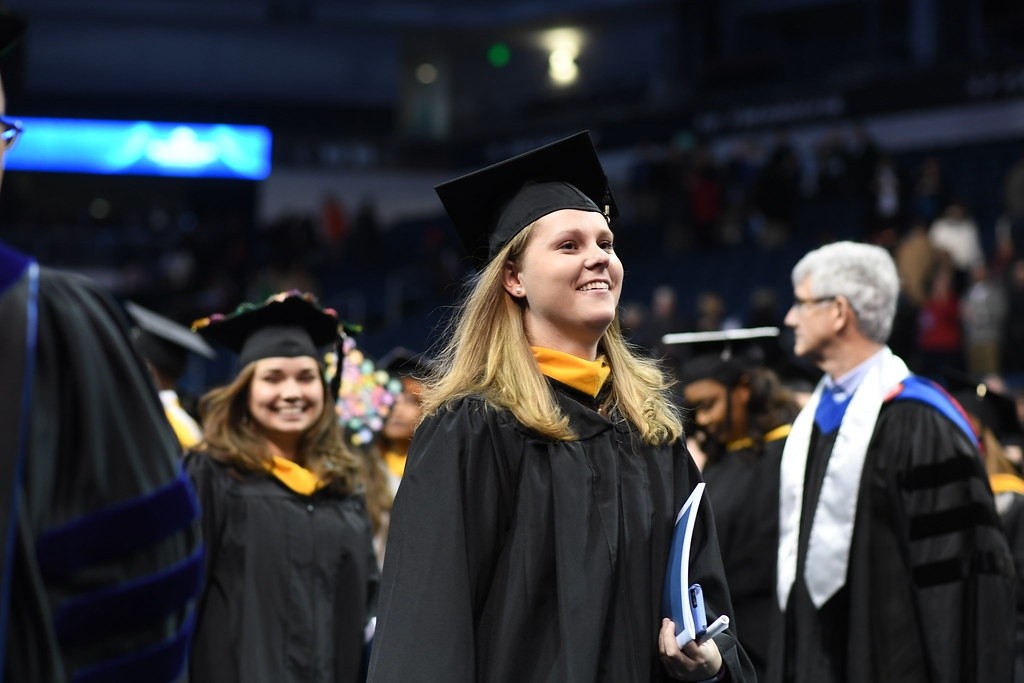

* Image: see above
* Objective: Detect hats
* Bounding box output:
[432,129,621,264]
[660,326,782,399]
[374,344,438,379]
[190,289,364,402]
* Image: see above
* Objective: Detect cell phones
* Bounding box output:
[688,583,707,639]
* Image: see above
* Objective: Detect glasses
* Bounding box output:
[789,295,841,313]
[0,114,27,152]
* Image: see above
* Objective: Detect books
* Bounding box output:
[662,483,706,650]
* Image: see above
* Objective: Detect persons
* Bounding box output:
[172,292,380,682]
[956,391,1023,566]
[0,93,200,682]
[374,348,432,573]
[308,136,1024,380]
[660,326,801,563]
[767,240,1023,683]
[366,128,758,683]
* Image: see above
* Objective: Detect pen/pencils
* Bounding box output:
[697,615,731,648]
